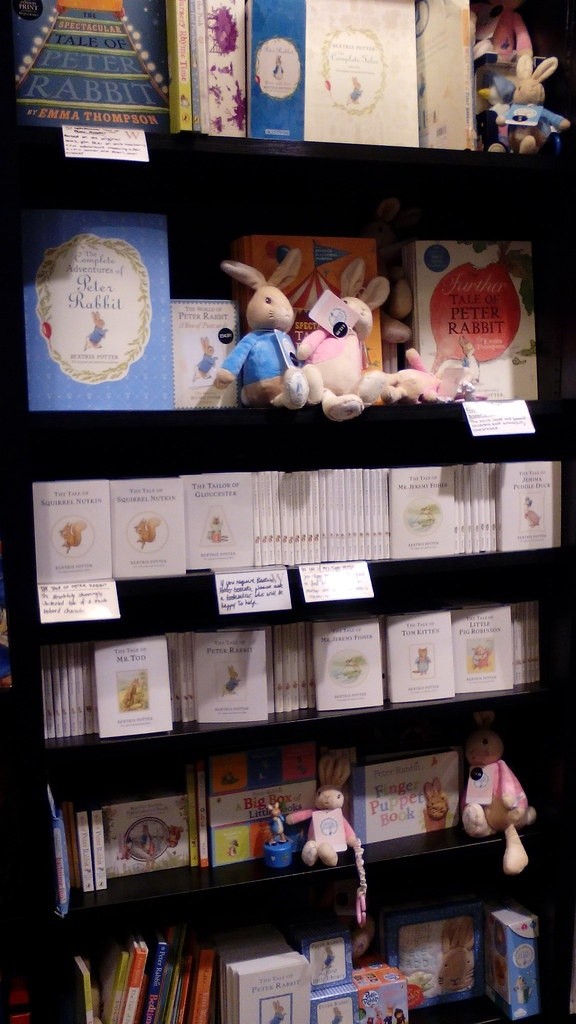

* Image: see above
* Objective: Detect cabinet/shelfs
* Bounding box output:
[0,0,576,1024]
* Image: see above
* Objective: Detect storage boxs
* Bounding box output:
[485,910,538,1020]
[282,904,409,1024]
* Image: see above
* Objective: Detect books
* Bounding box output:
[165,0,475,149]
[403,240,538,402]
[50,736,460,916]
[42,602,541,739]
[31,460,561,584]
[170,300,241,409]
[60,910,312,1024]
[21,207,173,410]
[235,235,385,404]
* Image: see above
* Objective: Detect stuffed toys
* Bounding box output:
[297,259,447,420]
[459,710,535,873]
[218,249,323,409]
[476,0,569,152]
[283,755,357,867]
